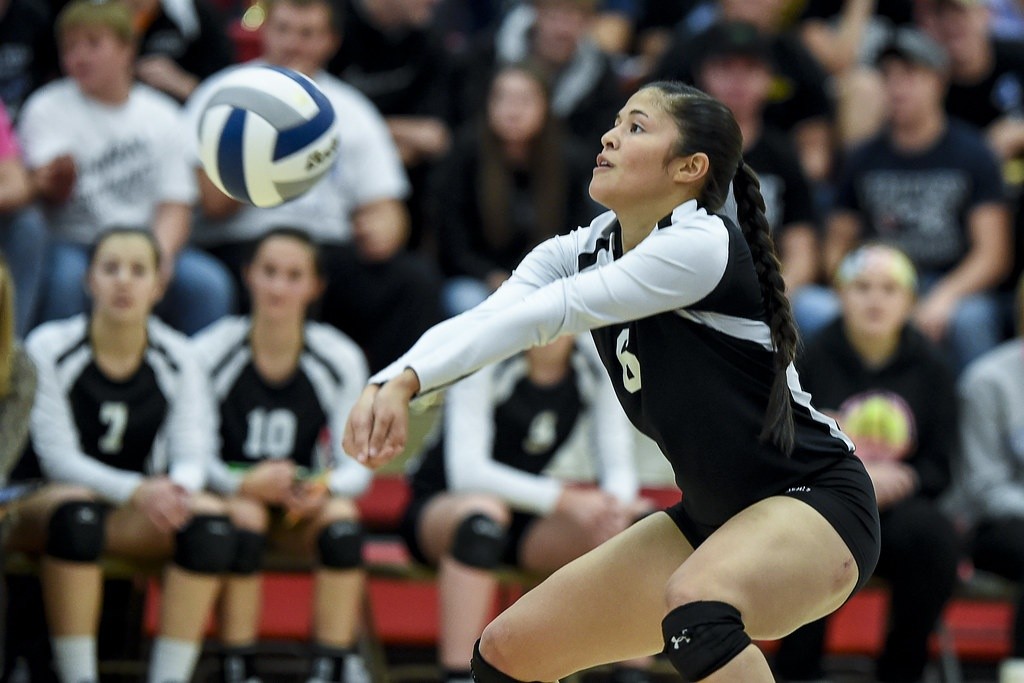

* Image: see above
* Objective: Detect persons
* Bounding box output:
[340,81,882,681]
[0,2,1024,683]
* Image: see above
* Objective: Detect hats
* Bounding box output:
[692,16,765,64]
[58,0,156,45]
[874,24,951,79]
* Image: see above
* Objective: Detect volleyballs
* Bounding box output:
[182,60,345,210]
